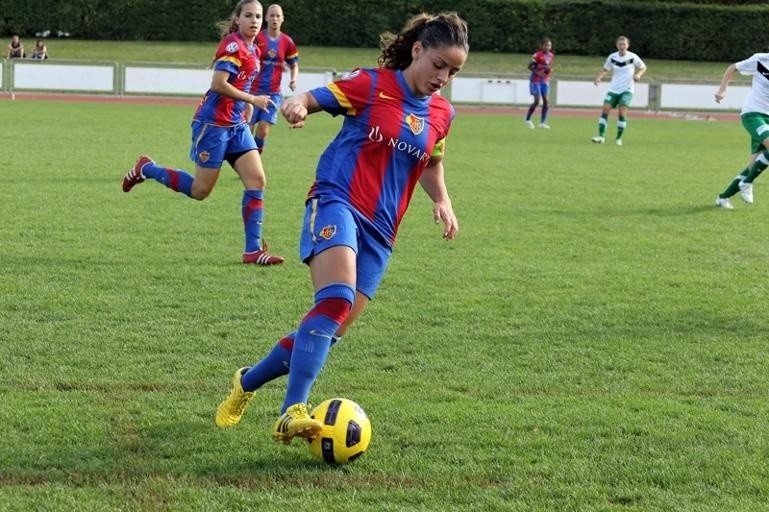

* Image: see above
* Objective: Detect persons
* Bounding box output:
[30,39,48,62]
[523,37,554,130]
[248,4,300,154]
[715,53,769,211]
[121,0,284,265]
[214,11,470,446]
[7,34,27,60]
[591,35,647,146]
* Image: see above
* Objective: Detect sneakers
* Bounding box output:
[738,178,754,205]
[591,135,606,145]
[522,115,535,130]
[614,137,623,146]
[715,194,735,209]
[538,122,551,130]
[270,403,323,448]
[213,365,257,431]
[122,154,156,193]
[240,238,285,267]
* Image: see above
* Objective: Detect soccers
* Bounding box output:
[309,398,370,463]
